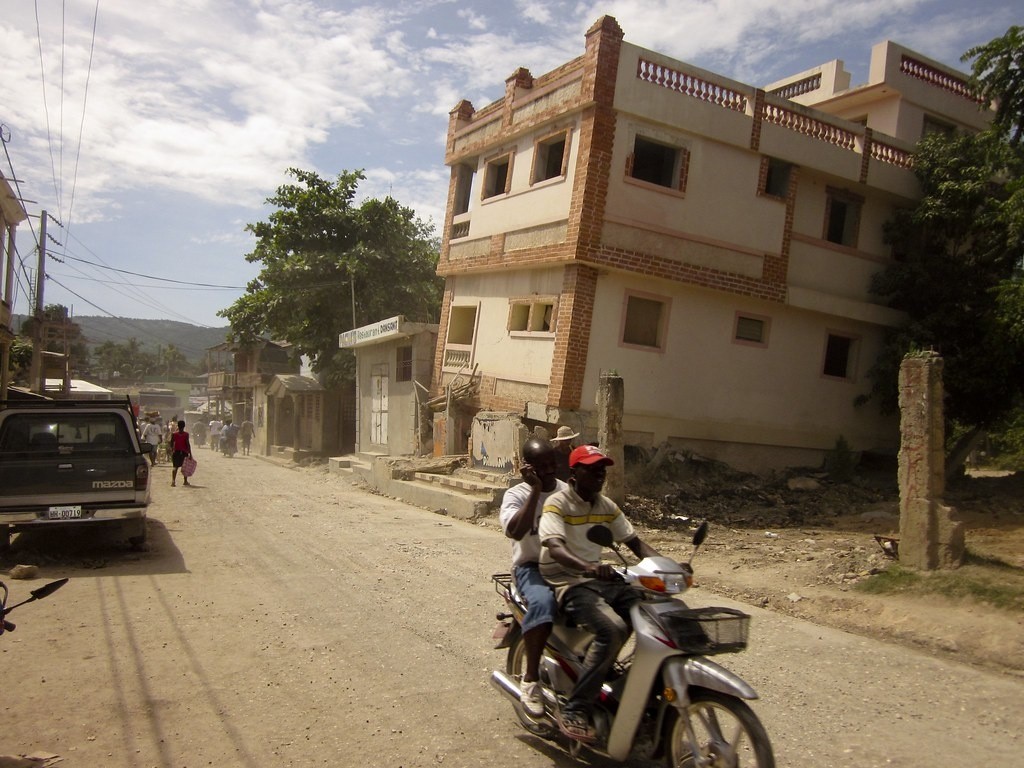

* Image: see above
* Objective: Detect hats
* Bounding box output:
[569,445,614,472]
[150,418,156,422]
[549,426,580,443]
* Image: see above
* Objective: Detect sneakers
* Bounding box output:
[520,675,544,717]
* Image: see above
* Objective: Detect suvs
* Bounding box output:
[0,395,153,557]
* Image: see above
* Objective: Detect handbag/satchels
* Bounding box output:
[181,457,197,476]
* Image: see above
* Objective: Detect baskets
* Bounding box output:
[658,606,751,655]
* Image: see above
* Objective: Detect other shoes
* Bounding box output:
[183,482,190,485]
[171,482,175,486]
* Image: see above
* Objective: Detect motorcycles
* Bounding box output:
[195,432,204,448]
[223,434,236,458]
[489,518,775,768]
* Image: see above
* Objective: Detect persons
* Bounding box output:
[193,418,206,449]
[137,414,178,466]
[499,439,567,717]
[538,444,694,741]
[209,416,256,457]
[549,426,580,467]
[170,421,191,487]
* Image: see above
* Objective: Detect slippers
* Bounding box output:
[554,710,597,743]
